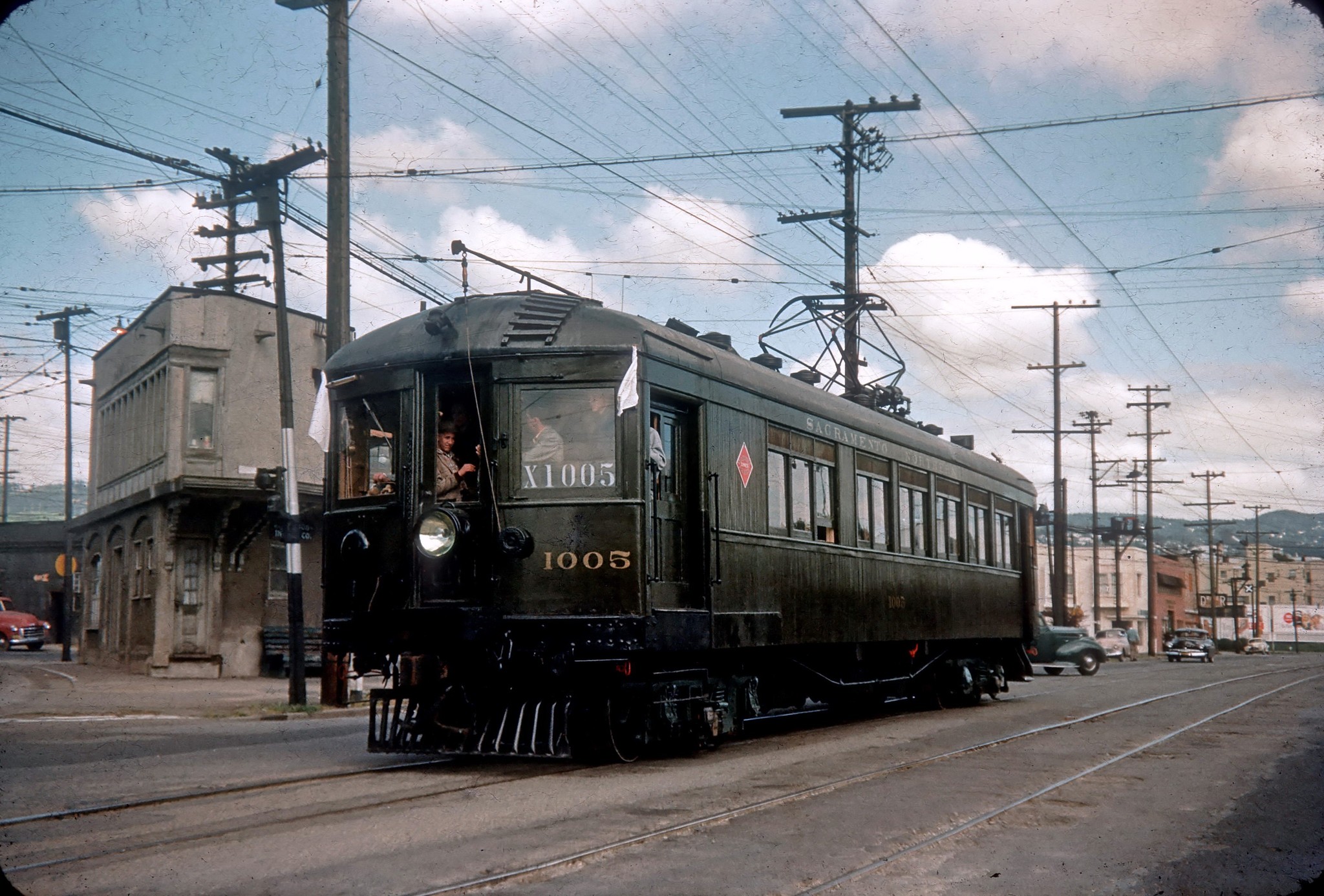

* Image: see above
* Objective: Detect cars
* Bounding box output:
[1243,638,1270,655]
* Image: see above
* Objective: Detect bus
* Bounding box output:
[319,288,1039,766]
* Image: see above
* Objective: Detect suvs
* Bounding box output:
[1026,611,1106,677]
[0,596,51,651]
[1162,627,1216,664]
[1095,628,1132,662]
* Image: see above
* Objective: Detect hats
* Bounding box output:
[437,421,458,434]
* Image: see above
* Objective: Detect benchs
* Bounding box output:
[261,625,321,678]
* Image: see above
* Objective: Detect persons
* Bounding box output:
[436,423,475,502]
[522,407,566,463]
[1127,623,1141,662]
[589,389,668,490]
